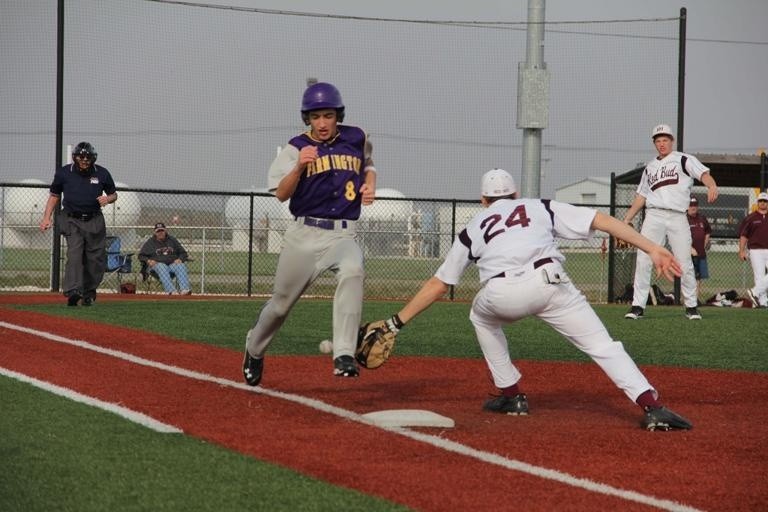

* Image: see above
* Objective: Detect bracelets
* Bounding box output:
[363,166,377,174]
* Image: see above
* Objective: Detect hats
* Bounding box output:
[481,169,516,197]
[756,192,768,202]
[651,124,673,139]
[154,223,166,232]
[690,196,698,205]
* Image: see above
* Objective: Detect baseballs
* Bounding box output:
[319,339,332,354]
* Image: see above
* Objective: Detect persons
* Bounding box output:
[139,221,195,297]
[354,168,697,431]
[685,197,713,309]
[40,141,119,306]
[616,123,721,323]
[242,81,378,387]
[738,193,767,308]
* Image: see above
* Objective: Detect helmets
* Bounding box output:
[301,83,344,125]
[72,143,98,170]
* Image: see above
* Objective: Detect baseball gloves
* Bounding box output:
[614,224,633,249]
[355,319,395,370]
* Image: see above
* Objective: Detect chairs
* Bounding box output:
[139,255,175,297]
[102,237,136,292]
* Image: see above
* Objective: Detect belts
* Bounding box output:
[294,216,347,230]
[491,256,552,278]
[64,210,100,221]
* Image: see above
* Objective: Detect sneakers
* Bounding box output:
[625,305,644,319]
[747,288,761,307]
[647,407,693,431]
[67,290,81,307]
[180,289,191,295]
[169,291,179,295]
[685,308,702,319]
[82,292,96,305]
[242,330,263,385]
[334,356,360,377]
[483,394,530,416]
